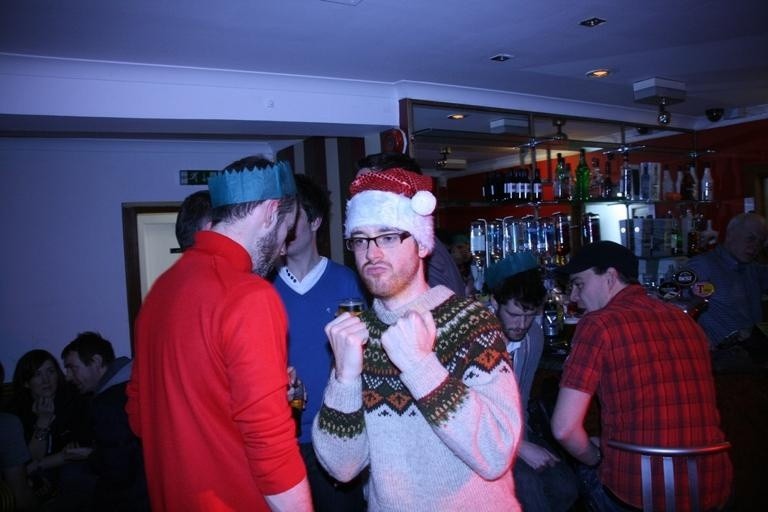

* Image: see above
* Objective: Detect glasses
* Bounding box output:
[344,231,411,253]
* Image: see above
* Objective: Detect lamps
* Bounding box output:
[551,117,568,139]
[431,147,451,171]
[657,97,671,126]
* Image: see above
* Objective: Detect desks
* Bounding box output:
[539,347,563,383]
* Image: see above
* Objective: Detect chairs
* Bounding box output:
[528,399,578,473]
[607,440,733,512]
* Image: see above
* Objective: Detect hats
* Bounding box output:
[345,169,437,251]
[559,241,637,275]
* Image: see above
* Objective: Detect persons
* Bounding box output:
[170,187,218,255]
[678,210,768,417]
[129,152,317,511]
[549,240,739,511]
[485,248,584,512]
[265,170,368,510]
[356,150,467,302]
[306,168,527,512]
[0,330,150,512]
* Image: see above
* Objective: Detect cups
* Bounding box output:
[337,299,364,316]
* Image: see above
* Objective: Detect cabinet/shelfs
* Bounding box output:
[400,97,718,258]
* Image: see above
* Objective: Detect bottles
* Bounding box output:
[287,381,305,437]
[641,163,676,202]
[485,165,543,201]
[675,162,715,201]
[555,150,634,200]
[633,209,718,258]
[470,213,599,268]
